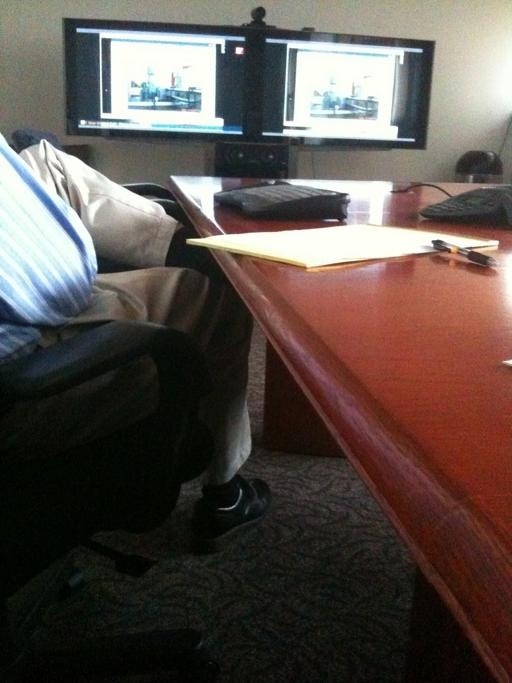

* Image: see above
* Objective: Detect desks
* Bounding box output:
[167,174,512,683]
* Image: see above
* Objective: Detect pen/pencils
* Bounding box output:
[432,240,500,267]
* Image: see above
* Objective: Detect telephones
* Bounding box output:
[420,186,512,228]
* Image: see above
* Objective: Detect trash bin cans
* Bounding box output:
[455,151,503,184]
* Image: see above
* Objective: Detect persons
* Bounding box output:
[0,130,274,554]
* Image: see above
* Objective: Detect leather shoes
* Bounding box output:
[191,477,271,554]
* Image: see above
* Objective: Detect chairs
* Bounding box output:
[0,181,227,682]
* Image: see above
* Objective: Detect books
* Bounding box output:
[183,222,501,271]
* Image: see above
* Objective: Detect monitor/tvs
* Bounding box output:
[255,28,435,149]
[62,18,255,142]
